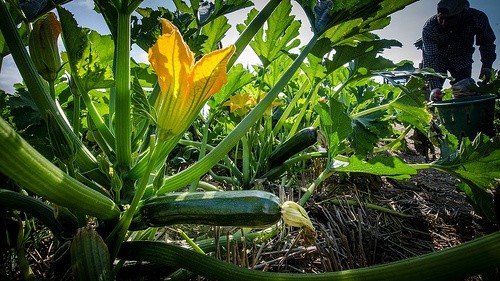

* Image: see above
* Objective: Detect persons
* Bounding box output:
[421,0,497,102]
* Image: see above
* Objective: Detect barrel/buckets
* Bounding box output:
[431,95,497,149]
